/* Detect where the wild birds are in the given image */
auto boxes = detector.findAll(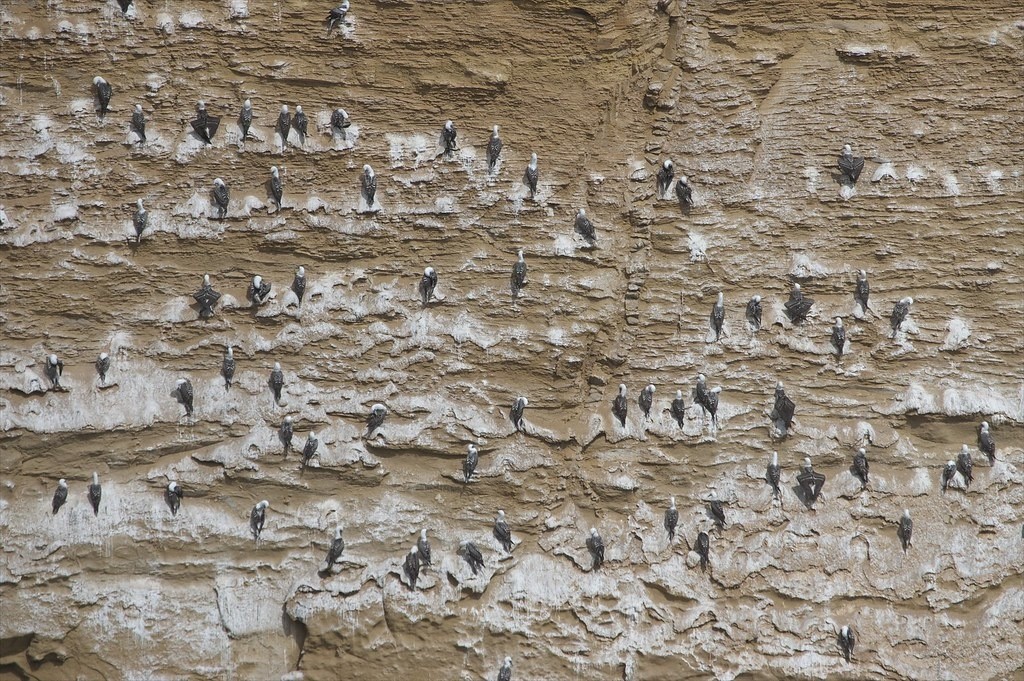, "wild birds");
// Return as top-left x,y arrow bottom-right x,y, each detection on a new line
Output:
325,0 -> 350,28
838,625 -> 855,662
498,656 -> 514,681
41,76 -> 996,593
